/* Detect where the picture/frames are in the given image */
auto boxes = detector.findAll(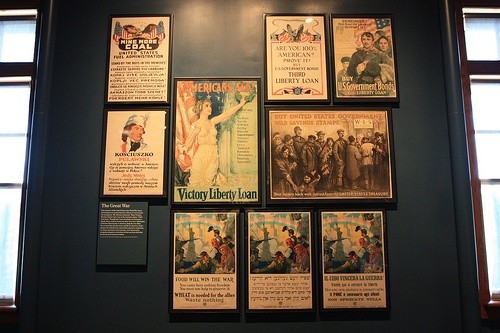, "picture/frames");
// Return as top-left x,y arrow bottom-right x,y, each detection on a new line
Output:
244,207 -> 317,314
263,105 -> 397,204
171,75 -> 262,207
103,13 -> 175,107
99,106 -> 171,199
169,208 -> 240,314
329,13 -> 401,105
317,208 -> 391,312
262,13 -> 332,105
